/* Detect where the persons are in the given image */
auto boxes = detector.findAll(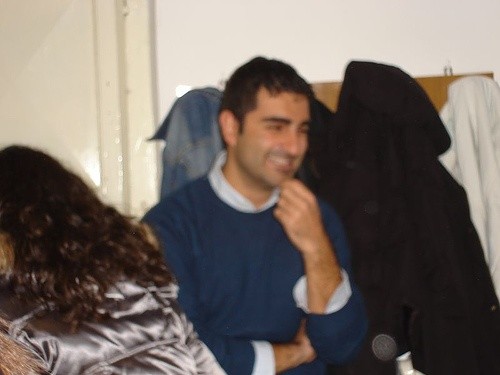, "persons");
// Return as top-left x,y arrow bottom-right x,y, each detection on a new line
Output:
135,56 -> 369,375
0,145 -> 228,375
0,327 -> 42,375
